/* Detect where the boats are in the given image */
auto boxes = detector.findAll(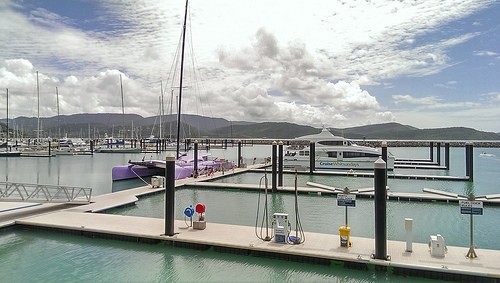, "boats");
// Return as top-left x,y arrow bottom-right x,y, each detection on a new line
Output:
280,138 -> 395,170
